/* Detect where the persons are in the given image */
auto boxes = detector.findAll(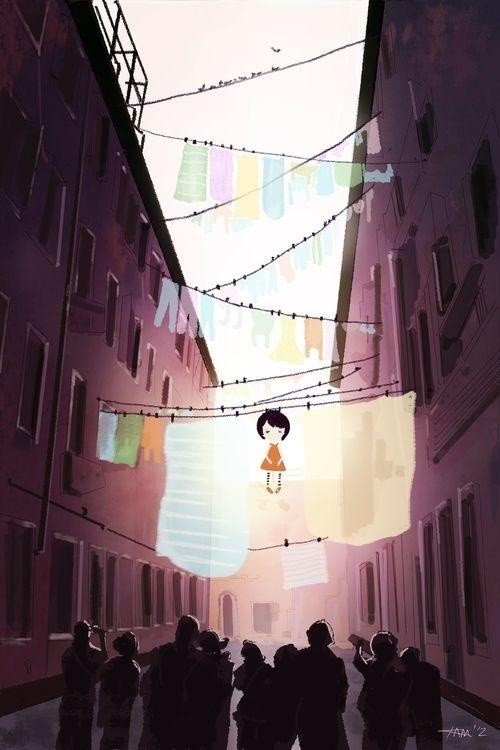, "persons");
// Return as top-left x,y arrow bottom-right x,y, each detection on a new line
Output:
59,614 -> 444,745
